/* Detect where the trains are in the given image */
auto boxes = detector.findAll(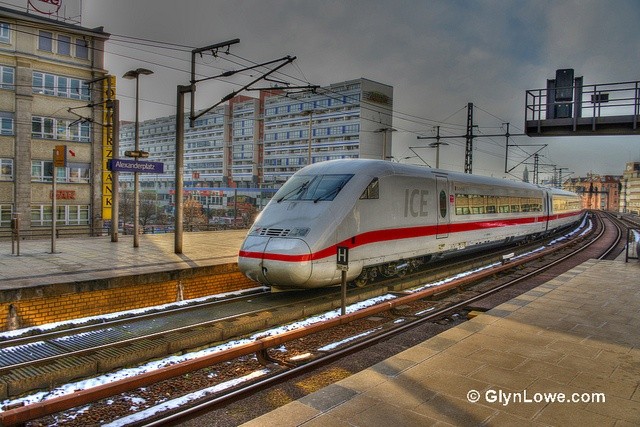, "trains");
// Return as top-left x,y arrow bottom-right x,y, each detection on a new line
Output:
238,158 -> 583,293
